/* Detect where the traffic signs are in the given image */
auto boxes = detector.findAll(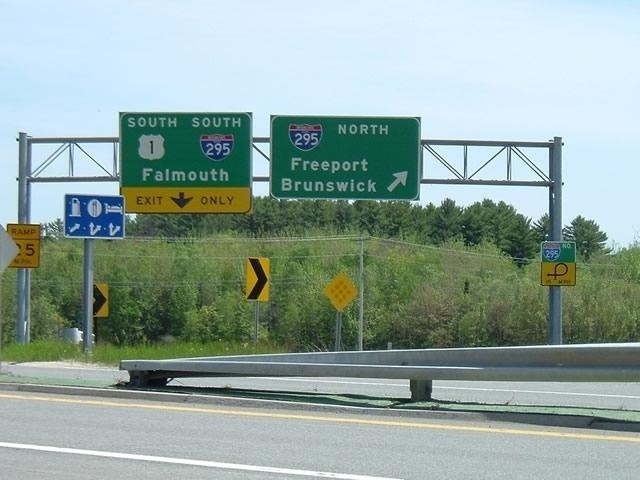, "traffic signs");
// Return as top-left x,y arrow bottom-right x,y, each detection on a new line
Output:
541,242 -> 576,262
5,223 -> 39,268
269,117 -> 421,200
541,262 -> 575,286
120,112 -> 252,186
